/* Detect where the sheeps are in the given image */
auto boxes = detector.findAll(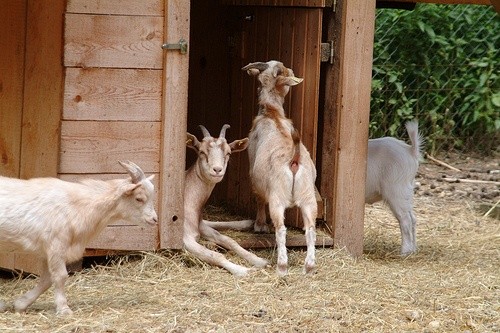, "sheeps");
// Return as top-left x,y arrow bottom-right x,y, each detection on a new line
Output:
1,160 -> 159,314
184,124 -> 267,278
364,117 -> 425,257
242,60 -> 318,276
